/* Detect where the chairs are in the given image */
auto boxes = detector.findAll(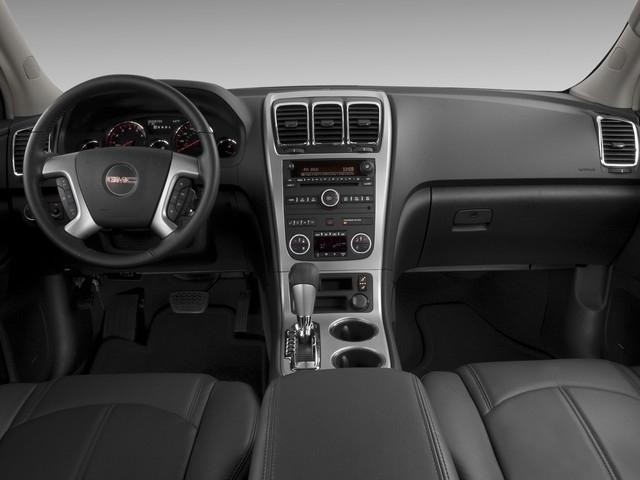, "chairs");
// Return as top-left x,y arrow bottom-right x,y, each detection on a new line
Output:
0,358 -> 256,478
418,354 -> 640,480
